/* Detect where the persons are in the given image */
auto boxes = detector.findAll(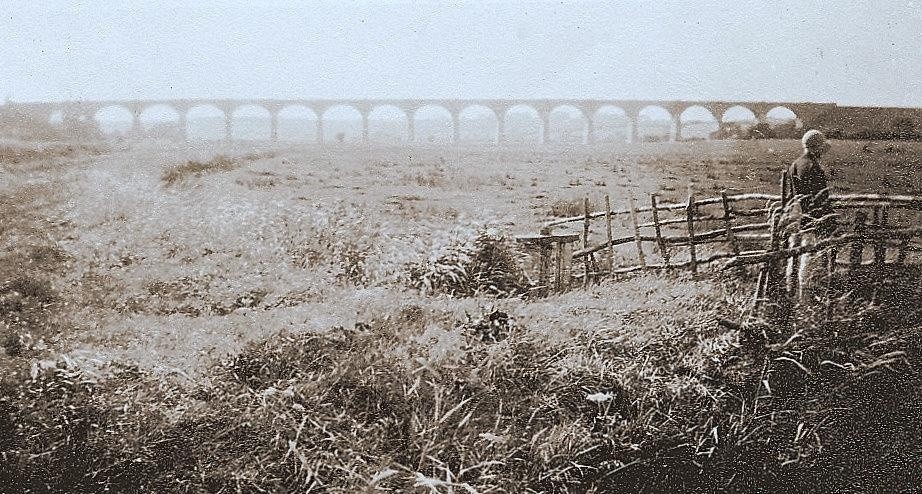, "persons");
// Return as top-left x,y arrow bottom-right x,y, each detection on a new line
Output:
786,129 -> 839,298
